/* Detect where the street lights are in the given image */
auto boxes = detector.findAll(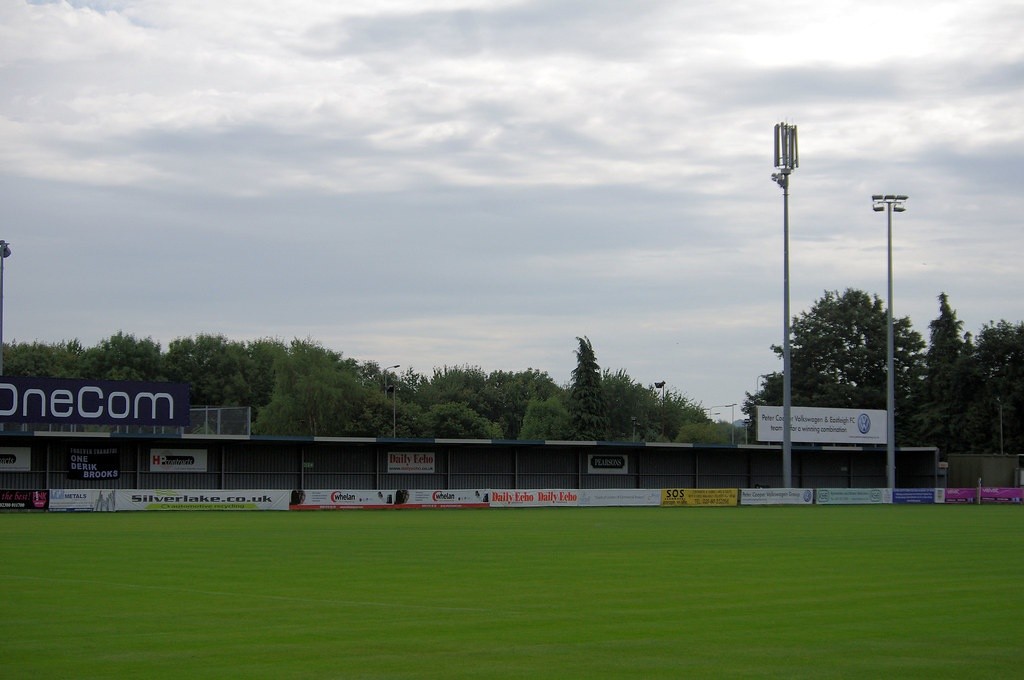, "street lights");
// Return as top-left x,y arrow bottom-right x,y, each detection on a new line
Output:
383,365 -> 399,398
725,404 -> 736,444
874,195 -> 908,489
